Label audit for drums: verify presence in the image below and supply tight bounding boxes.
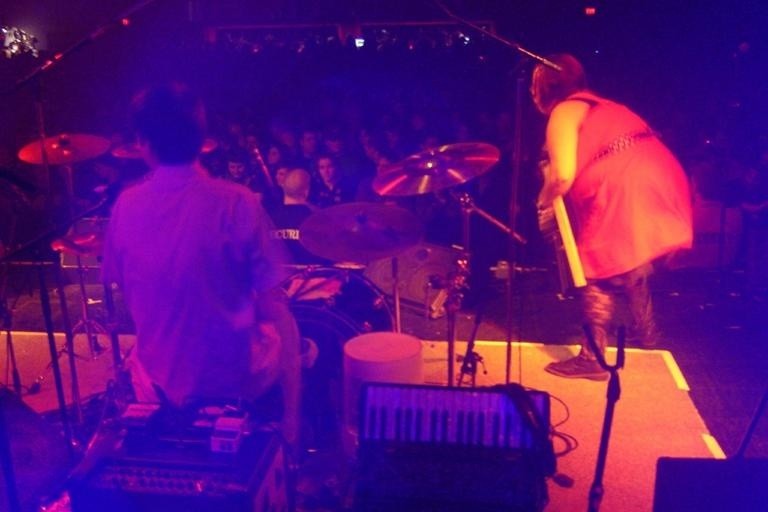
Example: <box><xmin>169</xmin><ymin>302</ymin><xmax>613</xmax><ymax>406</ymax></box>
<box><xmin>252</xmin><ymin>266</ymin><xmax>397</xmax><ymax>450</ymax></box>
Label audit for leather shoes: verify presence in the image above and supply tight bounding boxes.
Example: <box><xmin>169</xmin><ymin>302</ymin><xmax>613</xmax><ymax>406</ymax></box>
<box><xmin>545</xmin><ymin>356</ymin><xmax>609</xmax><ymax>381</ymax></box>
<box><xmin>613</xmin><ymin>324</ymin><xmax>659</xmax><ymax>347</ymax></box>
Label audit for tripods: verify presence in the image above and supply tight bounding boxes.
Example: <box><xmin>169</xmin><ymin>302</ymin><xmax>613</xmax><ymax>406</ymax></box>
<box><xmin>32</xmin><ymin>211</ymin><xmax>155</xmax><ymax>406</ymax></box>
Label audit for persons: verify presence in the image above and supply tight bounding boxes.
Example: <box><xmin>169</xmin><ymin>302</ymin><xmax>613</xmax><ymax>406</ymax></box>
<box><xmin>65</xmin><ymin>77</ymin><xmax>768</xmax><ymax>279</ymax></box>
<box><xmin>92</xmin><ymin>77</ymin><xmax>303</xmax><ymax>462</ymax></box>
<box><xmin>529</xmin><ymin>50</ymin><xmax>697</xmax><ymax>381</ymax></box>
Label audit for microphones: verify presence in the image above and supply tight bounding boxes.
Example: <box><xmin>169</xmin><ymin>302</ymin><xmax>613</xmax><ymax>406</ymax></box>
<box><xmin>90</xmin><ymin>1</ymin><xmax>141</xmax><ymax>46</ymax></box>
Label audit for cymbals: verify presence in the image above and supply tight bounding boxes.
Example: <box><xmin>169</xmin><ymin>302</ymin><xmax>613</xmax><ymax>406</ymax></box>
<box><xmin>298</xmin><ymin>201</ymin><xmax>425</xmax><ymax>263</ymax></box>
<box><xmin>17</xmin><ymin>133</ymin><xmax>111</xmax><ymax>165</ymax></box>
<box><xmin>371</xmin><ymin>141</ymin><xmax>503</xmax><ymax>197</ymax></box>
<box><xmin>112</xmin><ymin>137</ymin><xmax>218</xmax><ymax>159</ymax></box>
